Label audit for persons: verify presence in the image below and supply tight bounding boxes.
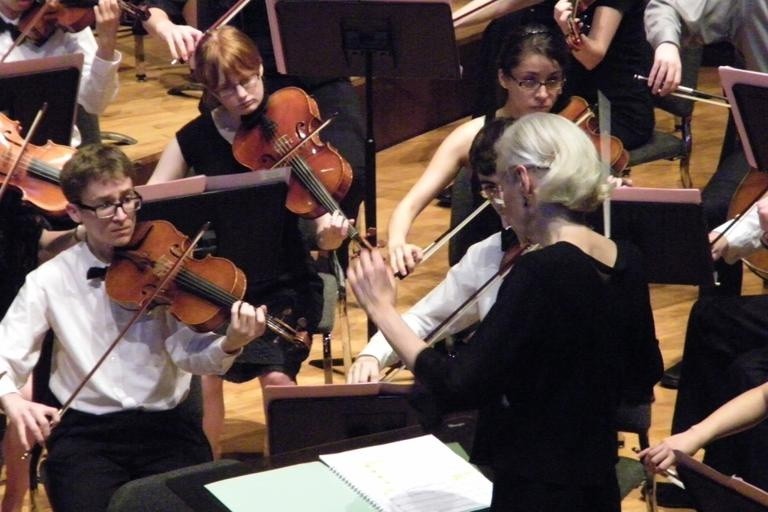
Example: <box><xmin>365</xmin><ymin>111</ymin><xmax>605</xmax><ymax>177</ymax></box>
<box><xmin>1</xmin><ymin>2</ymin><xmax>768</xmax><ymax>512</ymax></box>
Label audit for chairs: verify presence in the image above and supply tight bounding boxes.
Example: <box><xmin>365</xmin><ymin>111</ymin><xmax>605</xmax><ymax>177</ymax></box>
<box><xmin>621</xmin><ymin>45</ymin><xmax>700</xmax><ymax>189</ymax></box>
<box><xmin>308</xmin><ymin>249</ymin><xmax>341</xmax><ymax>387</ymax></box>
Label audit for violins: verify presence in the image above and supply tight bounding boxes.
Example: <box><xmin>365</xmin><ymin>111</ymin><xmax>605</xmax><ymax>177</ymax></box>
<box><xmin>551</xmin><ymin>95</ymin><xmax>630</xmax><ymax>175</ymax></box>
<box><xmin>19</xmin><ymin>1</ymin><xmax>150</xmax><ymax>42</ymax></box>
<box><xmin>105</xmin><ymin>219</ymin><xmax>312</xmax><ymax>350</ymax></box>
<box><xmin>0</xmin><ymin>111</ymin><xmax>78</xmax><ymax>217</ymax></box>
<box><xmin>231</xmin><ymin>86</ymin><xmax>373</xmax><ymax>249</ymax></box>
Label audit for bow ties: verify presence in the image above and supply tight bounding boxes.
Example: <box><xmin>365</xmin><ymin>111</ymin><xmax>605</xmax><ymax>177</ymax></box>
<box><xmin>0</xmin><ymin>18</ymin><xmax>24</xmax><ymax>43</ymax></box>
<box><xmin>87</xmin><ymin>267</ymin><xmax>109</xmax><ymax>279</ymax></box>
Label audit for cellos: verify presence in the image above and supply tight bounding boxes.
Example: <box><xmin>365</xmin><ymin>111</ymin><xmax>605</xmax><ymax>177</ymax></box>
<box><xmin>728</xmin><ymin>169</ymin><xmax>768</xmax><ymax>278</ymax></box>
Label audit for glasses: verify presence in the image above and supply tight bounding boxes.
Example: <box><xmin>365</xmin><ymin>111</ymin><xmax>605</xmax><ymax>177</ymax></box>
<box><xmin>510</xmin><ymin>76</ymin><xmax>566</xmax><ymax>88</ymax></box>
<box><xmin>78</xmin><ymin>192</ymin><xmax>142</xmax><ymax>218</ymax></box>
<box><xmin>212</xmin><ymin>73</ymin><xmax>259</xmax><ymax>97</ymax></box>
<box><xmin>479</xmin><ymin>184</ymin><xmax>502</xmax><ymax>199</ymax></box>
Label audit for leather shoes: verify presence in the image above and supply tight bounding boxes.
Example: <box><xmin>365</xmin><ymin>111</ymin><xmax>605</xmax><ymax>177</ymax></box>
<box><xmin>643</xmin><ymin>483</ymin><xmax>694</xmax><ymax>506</ymax></box>
<box><xmin>661</xmin><ymin>361</ymin><xmax>683</xmax><ymax>387</ymax></box>
